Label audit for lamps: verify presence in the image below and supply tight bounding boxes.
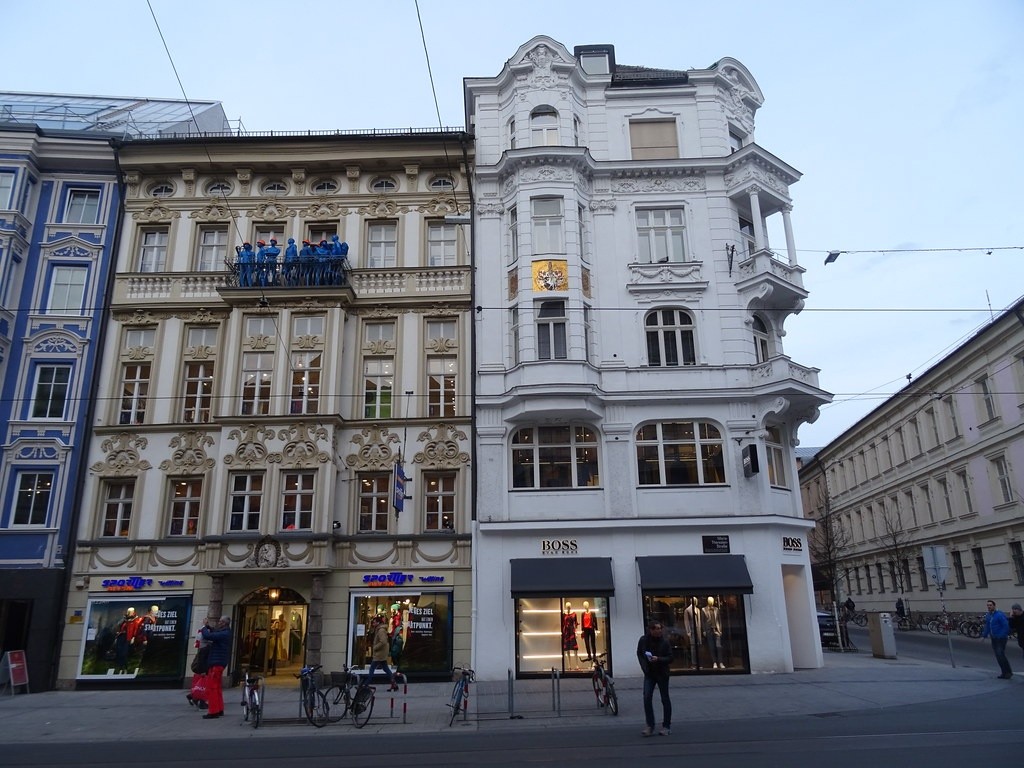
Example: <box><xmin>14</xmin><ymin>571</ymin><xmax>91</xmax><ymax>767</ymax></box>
<box><xmin>333</xmin><ymin>520</ymin><xmax>341</xmax><ymax>529</ymax></box>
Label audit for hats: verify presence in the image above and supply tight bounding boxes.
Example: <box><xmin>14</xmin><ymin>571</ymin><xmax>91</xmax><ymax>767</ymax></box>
<box><xmin>391</xmin><ymin>603</ymin><xmax>400</xmax><ymax>611</ymax></box>
<box><xmin>377</xmin><ymin>604</ymin><xmax>384</xmax><ymax>611</ymax></box>
<box><xmin>1012</xmin><ymin>603</ymin><xmax>1021</xmax><ymax>610</ymax></box>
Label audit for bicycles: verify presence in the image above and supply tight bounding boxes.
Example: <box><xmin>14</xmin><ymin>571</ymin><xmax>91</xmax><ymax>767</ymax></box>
<box><xmin>849</xmin><ymin>607</ymin><xmax>1018</xmax><ymax>640</ymax></box>
<box><xmin>446</xmin><ymin>662</ymin><xmax>477</xmax><ymax>728</ymax></box>
<box><xmin>323</xmin><ymin>663</ymin><xmax>376</xmax><ymax>729</ymax></box>
<box><xmin>292</xmin><ymin>662</ymin><xmax>330</xmax><ymax>728</ymax></box>
<box><xmin>232</xmin><ymin>665</ymin><xmax>261</xmax><ymax>729</ymax></box>
<box><xmin>580</xmin><ymin>648</ymin><xmax>619</xmax><ymax>717</ymax></box>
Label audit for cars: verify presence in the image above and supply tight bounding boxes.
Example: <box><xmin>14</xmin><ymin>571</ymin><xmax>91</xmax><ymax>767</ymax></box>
<box><xmin>815</xmin><ymin>609</ymin><xmax>849</xmax><ymax>647</ymax></box>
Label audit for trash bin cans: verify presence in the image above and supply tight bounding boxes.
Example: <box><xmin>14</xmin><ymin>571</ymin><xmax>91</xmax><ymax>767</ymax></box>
<box><xmin>867</xmin><ymin>611</ymin><xmax>897</xmax><ymax>659</ymax></box>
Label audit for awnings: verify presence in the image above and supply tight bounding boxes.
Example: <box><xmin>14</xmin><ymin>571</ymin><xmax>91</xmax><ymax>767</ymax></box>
<box><xmin>637</xmin><ymin>555</ymin><xmax>753</xmax><ymax>596</ymax></box>
<box><xmin>510</xmin><ymin>557</ymin><xmax>615</xmax><ymax>598</ymax></box>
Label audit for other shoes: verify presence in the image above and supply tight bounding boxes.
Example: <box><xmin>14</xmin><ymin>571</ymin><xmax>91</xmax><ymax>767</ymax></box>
<box><xmin>997</xmin><ymin>673</ymin><xmax>1012</xmax><ymax>679</ymax></box>
<box><xmin>187</xmin><ymin>694</ymin><xmax>193</xmax><ymax>705</ymax></box>
<box><xmin>387</xmin><ymin>686</ymin><xmax>399</xmax><ymax>692</ymax></box>
<box><xmin>203</xmin><ymin>713</ymin><xmax>219</xmax><ymax>718</ymax></box>
<box><xmin>200</xmin><ymin>704</ymin><xmax>208</xmax><ymax>709</ymax></box>
<box><xmin>219</xmin><ymin>711</ymin><xmax>224</xmax><ymax>716</ymax></box>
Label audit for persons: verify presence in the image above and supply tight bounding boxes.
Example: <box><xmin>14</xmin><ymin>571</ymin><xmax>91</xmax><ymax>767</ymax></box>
<box><xmin>367</xmin><ymin>617</ymin><xmax>399</xmax><ymax>692</ymax></box>
<box><xmin>701</xmin><ymin>597</ymin><xmax>725</xmax><ymax>669</ymax></box>
<box><xmin>185</xmin><ymin>616</ymin><xmax>232</xmax><ymax>719</ymax></box>
<box><xmin>1009</xmin><ymin>603</ymin><xmax>1024</xmax><ymax>649</ymax></box>
<box><xmin>896</xmin><ymin>598</ymin><xmax>906</xmax><ymax>626</ymax></box>
<box><xmin>637</xmin><ymin>620</ymin><xmax>673</xmax><ymax>735</ymax></box>
<box><xmin>239</xmin><ymin>235</ymin><xmax>348</xmax><ymax>287</ymax></box>
<box><xmin>115</xmin><ymin>605</ymin><xmax>159</xmax><ymax>674</ymax></box>
<box><xmin>563</xmin><ymin>602</ymin><xmax>580</xmax><ymax>670</ymax></box>
<box><xmin>581</xmin><ymin>601</ymin><xmax>598</xmax><ymax>658</ymax></box>
<box><xmin>684</xmin><ymin>597</ymin><xmax>703</xmax><ymax>669</ymax></box>
<box><xmin>845</xmin><ymin>597</ymin><xmax>855</xmax><ymax>621</ymax></box>
<box><xmin>979</xmin><ymin>600</ymin><xmax>1012</xmax><ymax>679</ymax></box>
<box><xmin>270</xmin><ymin>614</ymin><xmax>286</xmax><ymax>659</ymax></box>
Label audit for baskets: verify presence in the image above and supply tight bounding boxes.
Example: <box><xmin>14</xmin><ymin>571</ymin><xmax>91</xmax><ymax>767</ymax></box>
<box><xmin>331</xmin><ymin>671</ymin><xmax>346</xmax><ymax>686</ymax></box>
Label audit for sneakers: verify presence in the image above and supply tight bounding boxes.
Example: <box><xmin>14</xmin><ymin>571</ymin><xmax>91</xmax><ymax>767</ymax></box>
<box><xmin>659</xmin><ymin>728</ymin><xmax>672</xmax><ymax>736</ymax></box>
<box><xmin>642</xmin><ymin>726</ymin><xmax>654</xmax><ymax>736</ymax></box>
